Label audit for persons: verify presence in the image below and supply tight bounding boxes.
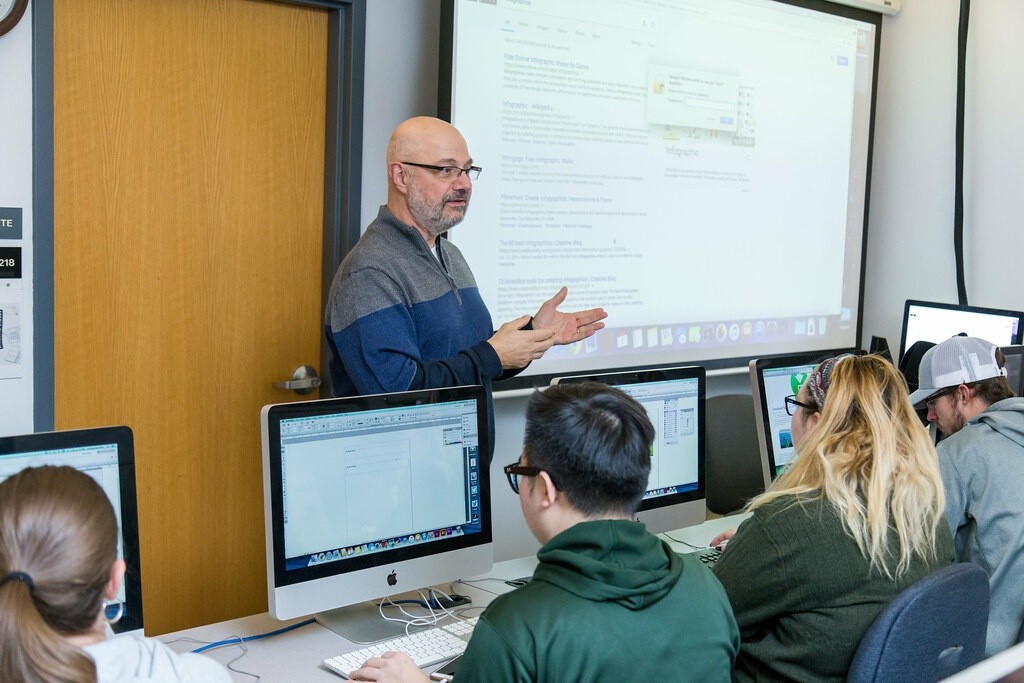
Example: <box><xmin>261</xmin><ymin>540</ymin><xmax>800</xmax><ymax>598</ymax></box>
<box><xmin>710</xmin><ymin>354</ymin><xmax>958</xmax><ymax>682</ymax></box>
<box><xmin>346</xmin><ymin>381</ymin><xmax>740</xmax><ymax>683</ymax></box>
<box><xmin>908</xmin><ymin>331</ymin><xmax>1024</xmax><ymax>656</ymax></box>
<box><xmin>323</xmin><ymin>115</ymin><xmax>608</xmax><ymax>397</ymax></box>
<box><xmin>0</xmin><ymin>464</ymin><xmax>235</xmax><ymax>682</ymax></box>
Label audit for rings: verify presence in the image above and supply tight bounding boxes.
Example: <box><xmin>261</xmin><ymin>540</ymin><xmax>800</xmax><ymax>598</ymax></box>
<box><xmin>577</xmin><ymin>329</ymin><xmax>579</xmax><ymax>333</ymax></box>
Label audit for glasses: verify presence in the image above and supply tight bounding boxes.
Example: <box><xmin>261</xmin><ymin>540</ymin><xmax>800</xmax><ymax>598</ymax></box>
<box><xmin>925</xmin><ymin>382</ymin><xmax>976</xmax><ymax>409</ymax></box>
<box><xmin>784</xmin><ymin>394</ymin><xmax>819</xmax><ymax>417</ymax></box>
<box><xmin>399</xmin><ymin>162</ymin><xmax>482</xmax><ymax>181</ymax></box>
<box><xmin>503</xmin><ymin>457</ymin><xmax>564</xmax><ymax>495</ymax></box>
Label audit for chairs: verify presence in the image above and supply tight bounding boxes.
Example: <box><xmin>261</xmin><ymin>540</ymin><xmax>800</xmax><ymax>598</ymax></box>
<box><xmin>845</xmin><ymin>562</ymin><xmax>990</xmax><ymax>683</ymax></box>
<box><xmin>703</xmin><ymin>394</ymin><xmax>765</xmax><ymax>514</ymax></box>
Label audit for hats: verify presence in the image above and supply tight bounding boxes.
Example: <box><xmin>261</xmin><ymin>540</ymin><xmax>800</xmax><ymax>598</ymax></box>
<box><xmin>905</xmin><ymin>334</ymin><xmax>1008</xmax><ymax>405</ymax></box>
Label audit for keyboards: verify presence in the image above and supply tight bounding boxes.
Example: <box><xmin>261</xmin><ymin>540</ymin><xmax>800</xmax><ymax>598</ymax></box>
<box><xmin>324</xmin><ymin>613</ymin><xmax>483</xmax><ymax>681</ymax></box>
<box><xmin>687</xmin><ymin>548</ymin><xmax>724</xmax><ymax>571</ymax></box>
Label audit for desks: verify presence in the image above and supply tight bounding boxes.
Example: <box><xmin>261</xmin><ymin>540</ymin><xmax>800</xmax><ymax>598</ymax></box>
<box><xmin>149</xmin><ymin>511</ymin><xmax>757</xmax><ymax>683</ymax></box>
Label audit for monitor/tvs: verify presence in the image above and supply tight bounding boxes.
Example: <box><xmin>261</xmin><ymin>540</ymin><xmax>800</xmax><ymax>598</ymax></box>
<box><xmin>550</xmin><ymin>365</ymin><xmax>707</xmax><ymax>536</ymax></box>
<box><xmin>260</xmin><ymin>384</ymin><xmax>494</xmax><ymax>645</ymax></box>
<box><xmin>749</xmin><ymin>350</ymin><xmax>869</xmax><ymax>491</ymax></box>
<box><xmin>898</xmin><ymin>300</ymin><xmax>1024</xmax><ymax>368</ymax></box>
<box><xmin>0</xmin><ymin>425</ymin><xmax>145</xmax><ymax>639</ymax></box>
